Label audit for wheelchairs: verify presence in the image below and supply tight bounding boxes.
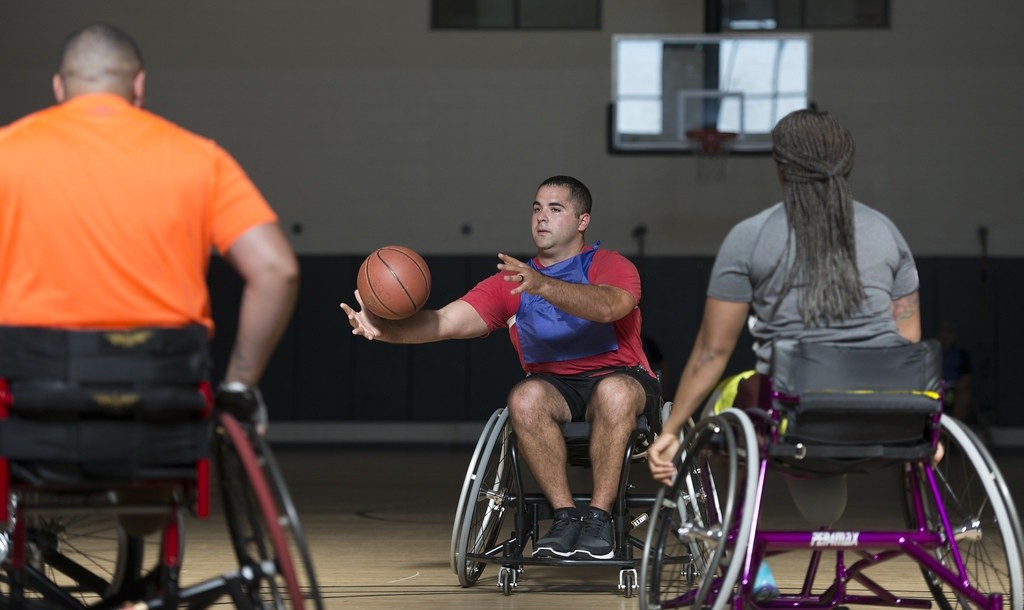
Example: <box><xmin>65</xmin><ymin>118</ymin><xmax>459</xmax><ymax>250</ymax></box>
<box><xmin>451</xmin><ymin>368</ymin><xmax>729</xmax><ymax>586</ymax></box>
<box><xmin>640</xmin><ymin>338</ymin><xmax>1024</xmax><ymax>610</ymax></box>
<box><xmin>0</xmin><ymin>321</ymin><xmax>326</xmax><ymax>610</ymax></box>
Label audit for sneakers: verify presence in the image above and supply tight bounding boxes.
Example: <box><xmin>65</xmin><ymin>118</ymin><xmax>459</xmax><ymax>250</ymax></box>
<box><xmin>744</xmin><ymin>557</ymin><xmax>781</xmax><ymax>600</ymax></box>
<box><xmin>568</xmin><ymin>512</ymin><xmax>618</xmax><ymax>560</ymax></box>
<box><xmin>531</xmin><ymin>508</ymin><xmax>582</xmax><ymax>559</ymax></box>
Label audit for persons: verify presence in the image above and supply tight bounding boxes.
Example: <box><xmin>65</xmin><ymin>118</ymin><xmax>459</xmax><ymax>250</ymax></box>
<box><xmin>642</xmin><ymin>106</ymin><xmax>924</xmax><ymax>596</ymax></box>
<box><xmin>0</xmin><ymin>18</ymin><xmax>298</xmax><ymax>432</ymax></box>
<box><xmin>338</xmin><ymin>174</ymin><xmax>665</xmax><ymax>567</ymax></box>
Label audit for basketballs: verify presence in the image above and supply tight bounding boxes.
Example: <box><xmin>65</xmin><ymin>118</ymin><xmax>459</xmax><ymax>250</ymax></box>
<box><xmin>357</xmin><ymin>245</ymin><xmax>432</xmax><ymax>321</ymax></box>
